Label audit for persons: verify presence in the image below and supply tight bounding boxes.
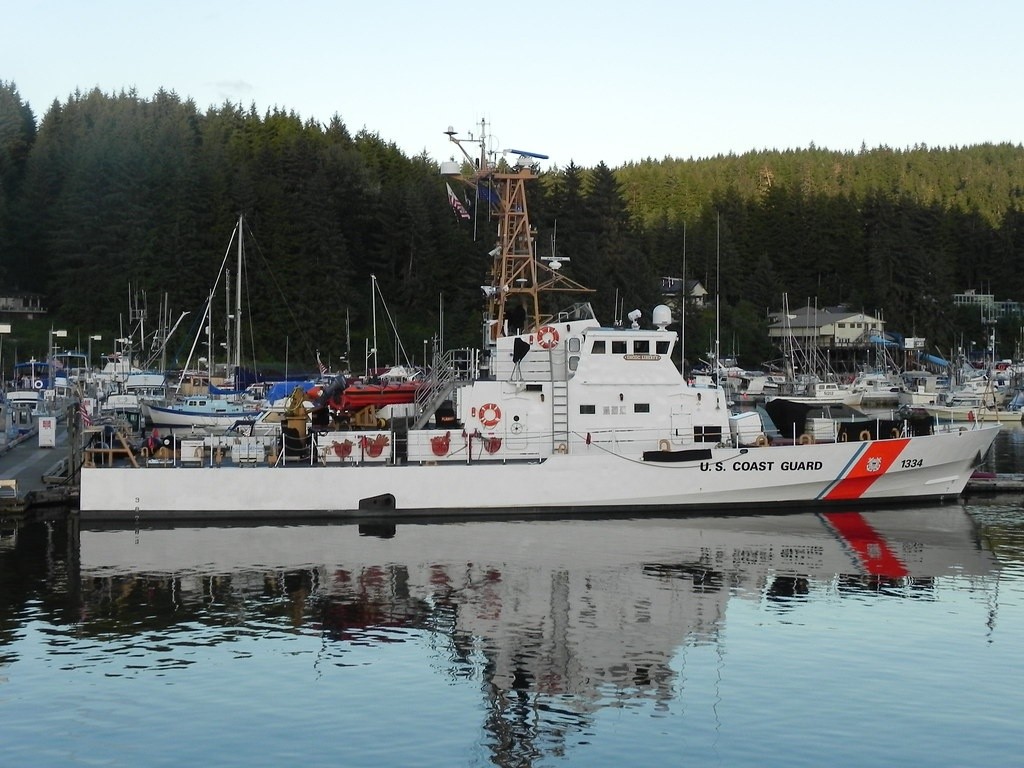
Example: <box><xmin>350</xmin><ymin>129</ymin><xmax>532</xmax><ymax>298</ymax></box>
<box><xmin>509</xmin><ymin>303</ymin><xmax>527</xmax><ymax>335</ymax></box>
<box><xmin>140</xmin><ymin>414</ymin><xmax>146</xmax><ymax>439</ymax></box>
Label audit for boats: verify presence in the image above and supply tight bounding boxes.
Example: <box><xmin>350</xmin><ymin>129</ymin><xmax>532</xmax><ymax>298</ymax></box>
<box><xmin>77</xmin><ymin>289</ymin><xmax>1005</xmax><ymax>523</ymax></box>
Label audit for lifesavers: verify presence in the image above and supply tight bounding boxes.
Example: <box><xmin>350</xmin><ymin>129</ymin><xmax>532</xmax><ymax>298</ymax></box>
<box><xmin>890</xmin><ymin>427</ymin><xmax>899</xmax><ymax>439</ymax></box>
<box><xmin>479</xmin><ymin>403</ymin><xmax>501</xmax><ymax>426</ymax></box>
<box><xmin>859</xmin><ymin>430</ymin><xmax>871</xmax><ymax>441</ymax></box>
<box><xmin>756</xmin><ymin>435</ymin><xmax>768</xmax><ymax>448</ymax></box>
<box><xmin>799</xmin><ymin>433</ymin><xmax>811</xmax><ymax>445</ymax></box>
<box><xmin>658</xmin><ymin>439</ymin><xmax>670</xmax><ymax>451</ymax></box>
<box><xmin>537</xmin><ymin>326</ymin><xmax>560</xmax><ymax>349</ymax></box>
<box><xmin>34</xmin><ymin>380</ymin><xmax>43</xmax><ymax>389</ymax></box>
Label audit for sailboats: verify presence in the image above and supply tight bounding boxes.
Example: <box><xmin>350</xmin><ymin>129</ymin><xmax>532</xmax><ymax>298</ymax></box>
<box><xmin>0</xmin><ymin>116</ymin><xmax>1023</xmax><ymax>488</ymax></box>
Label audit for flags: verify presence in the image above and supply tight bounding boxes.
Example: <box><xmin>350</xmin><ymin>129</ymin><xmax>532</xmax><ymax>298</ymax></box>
<box><xmin>78</xmin><ymin>394</ymin><xmax>93</xmax><ymax>426</ymax></box>
